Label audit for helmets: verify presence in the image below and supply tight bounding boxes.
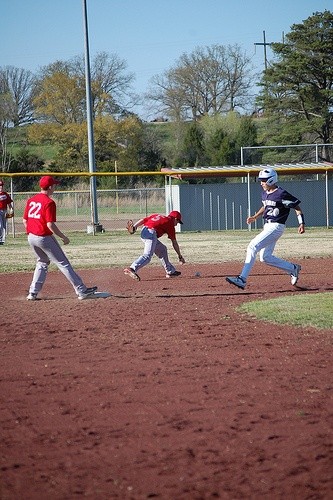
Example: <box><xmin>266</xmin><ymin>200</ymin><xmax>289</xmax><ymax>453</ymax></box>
<box><xmin>258</xmin><ymin>169</ymin><xmax>279</xmax><ymax>186</ymax></box>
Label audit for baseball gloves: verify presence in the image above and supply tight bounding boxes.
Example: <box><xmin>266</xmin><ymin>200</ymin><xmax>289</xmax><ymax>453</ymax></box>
<box><xmin>125</xmin><ymin>220</ymin><xmax>137</xmax><ymax>234</ymax></box>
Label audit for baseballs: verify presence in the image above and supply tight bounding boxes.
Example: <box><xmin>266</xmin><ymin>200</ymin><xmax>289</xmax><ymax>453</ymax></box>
<box><xmin>195</xmin><ymin>272</ymin><xmax>201</xmax><ymax>277</ymax></box>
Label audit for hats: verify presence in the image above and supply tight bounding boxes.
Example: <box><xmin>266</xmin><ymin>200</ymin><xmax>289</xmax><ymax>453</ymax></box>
<box><xmin>39</xmin><ymin>176</ymin><xmax>62</xmax><ymax>190</ymax></box>
<box><xmin>170</xmin><ymin>211</ymin><xmax>183</xmax><ymax>225</ymax></box>
<box><xmin>0</xmin><ymin>181</ymin><xmax>4</xmax><ymax>186</ymax></box>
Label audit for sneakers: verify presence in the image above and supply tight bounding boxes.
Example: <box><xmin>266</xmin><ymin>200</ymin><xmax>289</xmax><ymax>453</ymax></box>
<box><xmin>166</xmin><ymin>271</ymin><xmax>181</xmax><ymax>278</ymax></box>
<box><xmin>78</xmin><ymin>286</ymin><xmax>98</xmax><ymax>299</ymax></box>
<box><xmin>291</xmin><ymin>265</ymin><xmax>302</xmax><ymax>286</ymax></box>
<box><xmin>225</xmin><ymin>277</ymin><xmax>247</xmax><ymax>290</ymax></box>
<box><xmin>123</xmin><ymin>267</ymin><xmax>140</xmax><ymax>281</ymax></box>
<box><xmin>25</xmin><ymin>293</ymin><xmax>37</xmax><ymax>300</ymax></box>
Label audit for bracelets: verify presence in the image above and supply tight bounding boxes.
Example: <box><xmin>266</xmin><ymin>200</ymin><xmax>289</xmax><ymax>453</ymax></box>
<box><xmin>179</xmin><ymin>255</ymin><xmax>183</xmax><ymax>259</ymax></box>
<box><xmin>253</xmin><ymin>215</ymin><xmax>257</xmax><ymax>219</ymax></box>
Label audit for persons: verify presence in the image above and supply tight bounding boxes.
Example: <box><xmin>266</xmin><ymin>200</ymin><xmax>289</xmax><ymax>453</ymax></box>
<box><xmin>23</xmin><ymin>176</ymin><xmax>98</xmax><ymax>300</ymax></box>
<box><xmin>123</xmin><ymin>210</ymin><xmax>186</xmax><ymax>281</ymax></box>
<box><xmin>0</xmin><ymin>179</ymin><xmax>14</xmax><ymax>245</ymax></box>
<box><xmin>226</xmin><ymin>168</ymin><xmax>306</xmax><ymax>290</ymax></box>
